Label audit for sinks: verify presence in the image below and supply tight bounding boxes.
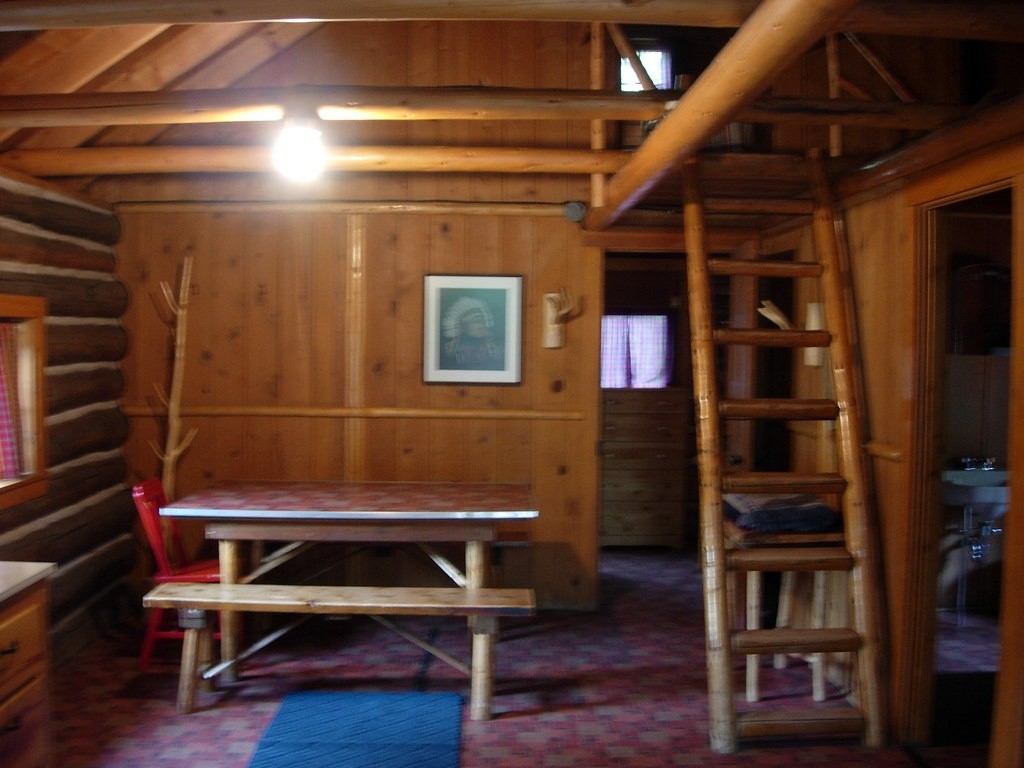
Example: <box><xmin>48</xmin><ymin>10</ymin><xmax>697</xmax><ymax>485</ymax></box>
<box><xmin>929</xmin><ymin>470</ymin><xmax>1011</xmax><ymax>522</ymax></box>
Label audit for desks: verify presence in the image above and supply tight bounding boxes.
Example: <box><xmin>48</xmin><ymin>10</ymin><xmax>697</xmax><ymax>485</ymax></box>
<box><xmin>158</xmin><ymin>481</ymin><xmax>542</xmax><ymax>683</ymax></box>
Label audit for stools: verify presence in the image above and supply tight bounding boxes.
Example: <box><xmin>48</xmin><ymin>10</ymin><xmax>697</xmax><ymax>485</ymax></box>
<box><xmin>720</xmin><ymin>494</ymin><xmax>847</xmax><ymax>704</ymax></box>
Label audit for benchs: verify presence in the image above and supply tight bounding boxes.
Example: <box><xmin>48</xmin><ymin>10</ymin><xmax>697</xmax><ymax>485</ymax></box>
<box><xmin>305</xmin><ymin>531</ymin><xmax>534</xmax><ymax>582</ymax></box>
<box><xmin>144</xmin><ymin>581</ymin><xmax>536</xmax><ymax>721</ymax></box>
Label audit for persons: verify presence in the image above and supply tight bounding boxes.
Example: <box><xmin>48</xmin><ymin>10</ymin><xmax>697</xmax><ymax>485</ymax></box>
<box><xmin>936</xmin><ymin>458</ymin><xmax>1005</xmax><ymax>601</ymax></box>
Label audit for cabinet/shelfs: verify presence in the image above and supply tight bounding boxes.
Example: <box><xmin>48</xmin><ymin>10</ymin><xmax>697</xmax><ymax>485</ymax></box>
<box><xmin>0</xmin><ymin>577</ymin><xmax>55</xmax><ymax>768</ymax></box>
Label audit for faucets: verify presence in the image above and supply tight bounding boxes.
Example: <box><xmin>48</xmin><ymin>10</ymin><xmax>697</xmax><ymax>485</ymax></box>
<box><xmin>959</xmin><ymin>454</ymin><xmax>998</xmax><ymax>472</ymax></box>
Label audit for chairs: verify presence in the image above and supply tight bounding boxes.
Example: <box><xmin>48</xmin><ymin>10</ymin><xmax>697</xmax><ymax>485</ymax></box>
<box><xmin>133</xmin><ymin>478</ymin><xmax>245</xmax><ymax>673</ymax></box>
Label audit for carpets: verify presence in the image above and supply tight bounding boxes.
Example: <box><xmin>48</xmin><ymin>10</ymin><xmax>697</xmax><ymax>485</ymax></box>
<box><xmin>247</xmin><ymin>689</ymin><xmax>463</xmax><ymax>768</ymax></box>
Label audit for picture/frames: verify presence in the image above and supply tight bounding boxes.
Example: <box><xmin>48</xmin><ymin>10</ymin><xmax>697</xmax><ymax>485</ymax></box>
<box><xmin>422</xmin><ymin>274</ymin><xmax>523</xmax><ymax>385</ymax></box>
